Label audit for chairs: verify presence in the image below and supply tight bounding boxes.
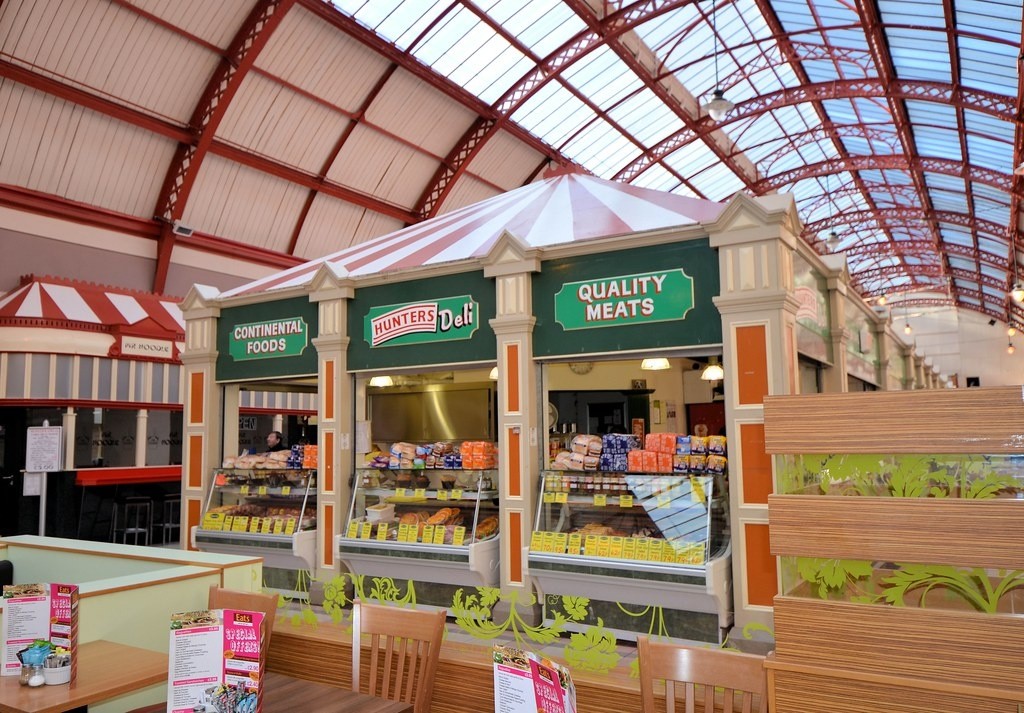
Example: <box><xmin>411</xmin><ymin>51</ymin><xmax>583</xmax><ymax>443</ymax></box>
<box><xmin>350</xmin><ymin>597</ymin><xmax>447</xmax><ymax>713</ymax></box>
<box><xmin>637</xmin><ymin>636</ymin><xmax>768</xmax><ymax>713</ymax></box>
<box><xmin>207</xmin><ymin>584</ymin><xmax>281</xmax><ymax>655</ymax></box>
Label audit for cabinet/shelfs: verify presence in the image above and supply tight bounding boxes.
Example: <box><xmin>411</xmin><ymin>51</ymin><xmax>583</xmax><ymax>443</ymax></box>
<box><xmin>194</xmin><ymin>466</ymin><xmax>316</xmax><ymax>600</ymax></box>
<box><xmin>334</xmin><ymin>466</ymin><xmax>500</xmax><ymax>622</ymax></box>
<box><xmin>527</xmin><ymin>469</ymin><xmax>733</xmax><ymax>650</ymax></box>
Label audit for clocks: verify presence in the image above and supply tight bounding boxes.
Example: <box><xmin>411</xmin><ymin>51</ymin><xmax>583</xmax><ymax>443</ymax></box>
<box><xmin>569</xmin><ymin>362</ymin><xmax>593</xmax><ymax>375</ymax></box>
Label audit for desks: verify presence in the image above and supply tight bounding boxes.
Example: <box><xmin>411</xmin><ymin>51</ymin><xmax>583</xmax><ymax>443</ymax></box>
<box><xmin>0</xmin><ymin>638</ymin><xmax>414</xmax><ymax>713</ymax></box>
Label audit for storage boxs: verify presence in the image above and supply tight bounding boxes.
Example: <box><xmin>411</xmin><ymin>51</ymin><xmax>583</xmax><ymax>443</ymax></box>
<box><xmin>598</xmin><ymin>433</ymin><xmax>691</xmax><ymax>473</ymax></box>
<box><xmin>152</xmin><ymin>523</ymin><xmax>180</xmax><ymax>544</ymax></box>
<box><xmin>115</xmin><ymin>529</ymin><xmax>150</xmax><ymax>547</ymax></box>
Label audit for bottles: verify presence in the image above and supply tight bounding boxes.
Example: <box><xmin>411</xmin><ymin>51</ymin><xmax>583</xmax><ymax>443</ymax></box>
<box><xmin>28</xmin><ymin>663</ymin><xmax>45</xmax><ymax>687</ymax></box>
<box><xmin>19</xmin><ymin>662</ymin><xmax>33</xmax><ymax>686</ymax></box>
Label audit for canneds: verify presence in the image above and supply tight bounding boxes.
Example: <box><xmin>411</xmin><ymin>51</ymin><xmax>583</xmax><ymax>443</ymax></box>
<box><xmin>544</xmin><ymin>475</ymin><xmax>693</xmax><ymax>499</ymax></box>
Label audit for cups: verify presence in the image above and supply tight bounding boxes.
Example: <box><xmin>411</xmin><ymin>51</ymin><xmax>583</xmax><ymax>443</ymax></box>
<box><xmin>43</xmin><ymin>665</ymin><xmax>71</xmax><ymax>685</ymax></box>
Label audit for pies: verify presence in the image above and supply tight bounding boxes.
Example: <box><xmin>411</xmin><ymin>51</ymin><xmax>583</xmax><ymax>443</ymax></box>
<box><xmin>399</xmin><ymin>507</ymin><xmax>498</xmax><ymax>538</ymax></box>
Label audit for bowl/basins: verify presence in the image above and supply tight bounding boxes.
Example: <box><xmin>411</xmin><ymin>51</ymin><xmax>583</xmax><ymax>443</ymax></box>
<box><xmin>365</xmin><ymin>503</ymin><xmax>395</xmax><ymax>525</ymax></box>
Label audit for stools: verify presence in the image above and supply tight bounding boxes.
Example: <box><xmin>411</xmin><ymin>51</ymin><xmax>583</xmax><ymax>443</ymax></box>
<box><xmin>162</xmin><ymin>494</ymin><xmax>181</xmax><ymax>545</ymax></box>
<box><xmin>108</xmin><ymin>496</ymin><xmax>153</xmax><ymax>546</ymax></box>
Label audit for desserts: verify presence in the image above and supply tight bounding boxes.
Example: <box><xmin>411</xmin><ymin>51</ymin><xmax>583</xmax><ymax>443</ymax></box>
<box><xmin>393</xmin><ymin>473</ymin><xmax>456</xmax><ymax>488</ymax></box>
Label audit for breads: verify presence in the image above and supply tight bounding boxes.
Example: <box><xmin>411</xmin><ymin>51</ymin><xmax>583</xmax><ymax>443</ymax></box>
<box><xmin>222</xmin><ymin>450</ymin><xmax>291</xmax><ymax>469</ymax></box>
<box><xmin>206</xmin><ymin>503</ymin><xmax>317</xmax><ymax>532</ymax></box>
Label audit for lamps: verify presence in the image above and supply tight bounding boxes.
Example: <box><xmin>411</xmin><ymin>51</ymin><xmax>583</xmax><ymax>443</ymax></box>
<box><xmin>640</xmin><ymin>358</ymin><xmax>671</xmax><ymax>371</ymax></box>
<box><xmin>824</xmin><ymin>175</ymin><xmax>844</xmax><ymax>249</ymax></box>
<box><xmin>488</xmin><ymin>367</ymin><xmax>499</xmax><ymax>380</ymax></box>
<box><xmin>1007</xmin><ymin>231</ymin><xmax>1024</xmax><ymax>304</ymax></box>
<box><xmin>369</xmin><ymin>376</ymin><xmax>394</xmax><ymax>389</ymax></box>
<box><xmin>153</xmin><ymin>216</ymin><xmax>195</xmax><ymax>238</ymax></box>
<box><xmin>1006</xmin><ymin>311</ymin><xmax>1016</xmax><ymax>337</ymax></box>
<box><xmin>1006</xmin><ymin>337</ymin><xmax>1016</xmax><ymax>355</ymax></box>
<box><xmin>699</xmin><ymin>357</ymin><xmax>724</xmax><ymax>381</ymax></box>
<box><xmin>904</xmin><ymin>292</ymin><xmax>912</xmax><ymax>334</ymax></box>
<box><xmin>702</xmin><ymin>0</ymin><xmax>736</xmax><ymax>121</ymax></box>
<box><xmin>878</xmin><ymin>253</ymin><xmax>888</xmax><ymax>305</ymax></box>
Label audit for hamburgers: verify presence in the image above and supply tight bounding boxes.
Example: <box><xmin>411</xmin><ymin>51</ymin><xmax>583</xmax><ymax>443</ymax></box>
<box><xmin>182</xmin><ymin>610</ymin><xmax>220</xmax><ymax>628</ymax></box>
<box><xmin>13</xmin><ymin>584</ymin><xmax>46</xmax><ymax>597</ymax></box>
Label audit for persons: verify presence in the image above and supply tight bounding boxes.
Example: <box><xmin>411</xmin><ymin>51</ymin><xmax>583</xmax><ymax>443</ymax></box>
<box><xmin>266</xmin><ymin>431</ymin><xmax>288</xmax><ymax>452</ymax></box>
<box><xmin>299</xmin><ymin>437</ymin><xmax>310</xmax><ymax>445</ymax></box>
